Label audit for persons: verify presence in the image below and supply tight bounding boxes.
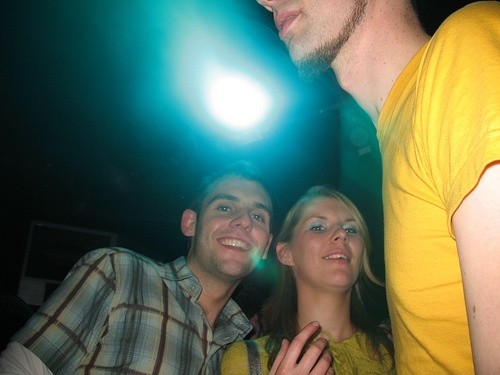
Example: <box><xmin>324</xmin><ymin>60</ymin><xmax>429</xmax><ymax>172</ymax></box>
<box><xmin>256</xmin><ymin>0</ymin><xmax>500</xmax><ymax>375</ymax></box>
<box><xmin>221</xmin><ymin>184</ymin><xmax>396</xmax><ymax>374</ymax></box>
<box><xmin>0</xmin><ymin>162</ymin><xmax>272</xmax><ymax>375</ymax></box>
